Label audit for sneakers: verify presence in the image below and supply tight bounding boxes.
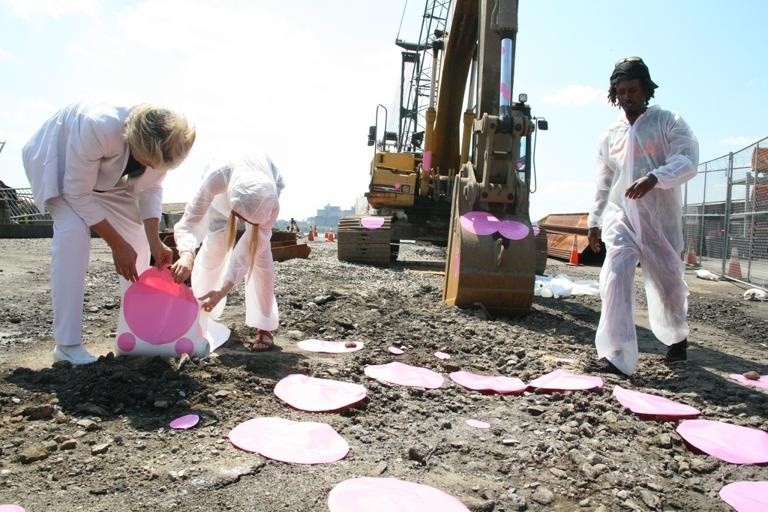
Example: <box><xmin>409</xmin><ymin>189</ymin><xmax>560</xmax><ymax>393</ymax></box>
<box><xmin>583</xmin><ymin>357</ymin><xmax>620</xmax><ymax>373</ymax></box>
<box><xmin>665</xmin><ymin>340</ymin><xmax>688</xmax><ymax>364</ymax></box>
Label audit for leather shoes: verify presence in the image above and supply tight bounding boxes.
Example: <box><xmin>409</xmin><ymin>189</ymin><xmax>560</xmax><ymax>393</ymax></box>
<box><xmin>53</xmin><ymin>343</ymin><xmax>97</xmax><ymax>366</ymax></box>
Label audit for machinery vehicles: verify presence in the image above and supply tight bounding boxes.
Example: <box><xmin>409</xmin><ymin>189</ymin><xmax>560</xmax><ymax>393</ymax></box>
<box><xmin>337</xmin><ymin>0</ymin><xmax>547</xmax><ymax>316</ymax></box>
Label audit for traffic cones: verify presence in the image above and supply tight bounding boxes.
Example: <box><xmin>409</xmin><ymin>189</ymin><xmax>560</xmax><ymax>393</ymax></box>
<box><xmin>566</xmin><ymin>235</ymin><xmax>582</xmax><ymax>265</ymax></box>
<box><xmin>309</xmin><ymin>224</ymin><xmax>317</xmax><ymax>241</ymax></box>
<box><xmin>324</xmin><ymin>225</ymin><xmax>333</xmax><ymax>241</ymax></box>
<box><xmin>728</xmin><ymin>246</ymin><xmax>744</xmax><ymax>280</ymax></box>
<box><xmin>683</xmin><ymin>235</ymin><xmax>700</xmax><ymax>267</ymax></box>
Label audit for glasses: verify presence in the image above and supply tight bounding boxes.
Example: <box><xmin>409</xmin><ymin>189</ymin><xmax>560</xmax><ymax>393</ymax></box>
<box><xmin>615</xmin><ymin>55</ymin><xmax>641</xmax><ymax>66</ymax></box>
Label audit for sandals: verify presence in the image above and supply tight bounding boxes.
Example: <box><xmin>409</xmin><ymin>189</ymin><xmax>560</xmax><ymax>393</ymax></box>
<box><xmin>253</xmin><ymin>330</ymin><xmax>274</xmax><ymax>353</ymax></box>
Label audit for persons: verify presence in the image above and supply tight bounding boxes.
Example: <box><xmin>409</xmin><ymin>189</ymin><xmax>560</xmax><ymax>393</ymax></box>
<box><xmin>581</xmin><ymin>57</ymin><xmax>698</xmax><ymax>377</ymax></box>
<box><xmin>22</xmin><ymin>99</ymin><xmax>197</xmax><ymax>366</ymax></box>
<box><xmin>171</xmin><ymin>154</ymin><xmax>287</xmax><ymax>351</ymax></box>
